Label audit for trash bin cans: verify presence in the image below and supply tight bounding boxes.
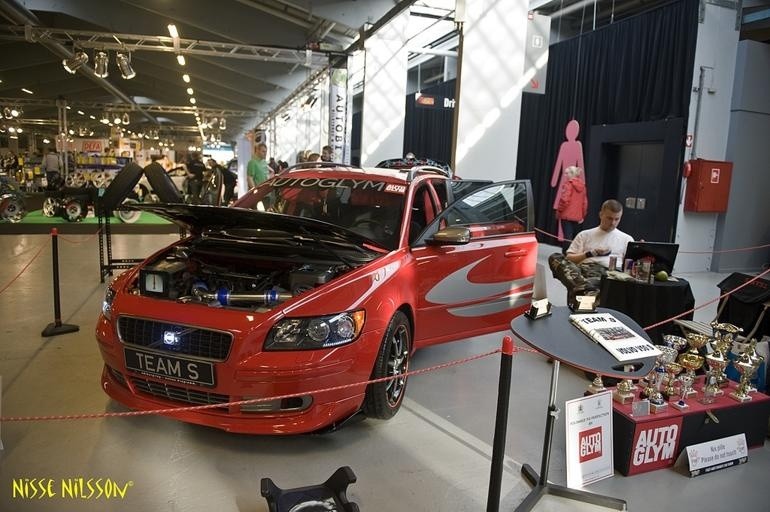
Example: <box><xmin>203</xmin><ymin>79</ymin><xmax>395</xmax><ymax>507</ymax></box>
<box><xmin>716</xmin><ymin>271</ymin><xmax>770</xmax><ymax>342</ymax></box>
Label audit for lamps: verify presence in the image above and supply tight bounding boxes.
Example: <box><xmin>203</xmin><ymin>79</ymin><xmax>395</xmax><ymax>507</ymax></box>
<box><xmin>61</xmin><ymin>39</ymin><xmax>137</xmax><ymax>80</ymax></box>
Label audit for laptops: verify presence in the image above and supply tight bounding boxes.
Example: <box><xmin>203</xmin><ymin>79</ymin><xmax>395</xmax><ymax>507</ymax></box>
<box><xmin>605</xmin><ymin>241</ymin><xmax>679</xmax><ymax>277</ymax></box>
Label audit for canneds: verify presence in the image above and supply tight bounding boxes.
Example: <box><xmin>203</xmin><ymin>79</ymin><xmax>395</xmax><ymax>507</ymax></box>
<box><xmin>624</xmin><ymin>258</ymin><xmax>633</xmax><ymax>274</ymax></box>
<box><xmin>609</xmin><ymin>255</ymin><xmax>616</xmax><ymax>270</ymax></box>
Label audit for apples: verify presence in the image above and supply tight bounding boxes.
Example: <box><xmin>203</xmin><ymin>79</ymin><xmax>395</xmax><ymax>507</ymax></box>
<box><xmin>656</xmin><ymin>271</ymin><xmax>668</xmax><ymax>281</ymax></box>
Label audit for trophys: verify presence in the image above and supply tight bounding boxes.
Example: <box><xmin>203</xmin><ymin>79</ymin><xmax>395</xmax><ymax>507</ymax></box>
<box><xmin>588</xmin><ymin>321</ymin><xmax>763</xmax><ymax>414</ymax></box>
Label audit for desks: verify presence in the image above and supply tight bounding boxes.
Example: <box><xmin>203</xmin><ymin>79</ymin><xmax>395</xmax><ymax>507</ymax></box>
<box><xmin>509</xmin><ymin>269</ymin><xmax>693</xmax><ymax>512</ymax></box>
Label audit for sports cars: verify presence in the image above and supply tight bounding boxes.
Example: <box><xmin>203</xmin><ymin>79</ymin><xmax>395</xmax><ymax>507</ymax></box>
<box><xmin>95</xmin><ymin>159</ymin><xmax>537</xmax><ymax>439</ymax></box>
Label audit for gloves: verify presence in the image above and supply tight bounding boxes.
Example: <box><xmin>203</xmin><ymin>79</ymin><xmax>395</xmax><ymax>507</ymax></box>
<box><xmin>586</xmin><ymin>247</ymin><xmax>611</xmax><ymax>258</ymax></box>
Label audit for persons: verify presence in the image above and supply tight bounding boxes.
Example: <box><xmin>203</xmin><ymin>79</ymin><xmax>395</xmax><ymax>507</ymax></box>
<box><xmin>42</xmin><ymin>148</ymin><xmax>63</xmax><ymax>186</ymax></box>
<box><xmin>548</xmin><ymin>200</ymin><xmax>636</xmax><ymax>305</ymax></box>
<box><xmin>190</xmin><ymin>152</ymin><xmax>237</xmax><ymax>206</ymax></box>
<box><xmin>558</xmin><ymin>166</ymin><xmax>588</xmax><ymax>255</ymax></box>
<box><xmin>5</xmin><ymin>151</ymin><xmax>18</xmax><ymax>174</ymax></box>
<box><xmin>247</xmin><ymin>144</ymin><xmax>332</xmax><ymax>210</ymax></box>
